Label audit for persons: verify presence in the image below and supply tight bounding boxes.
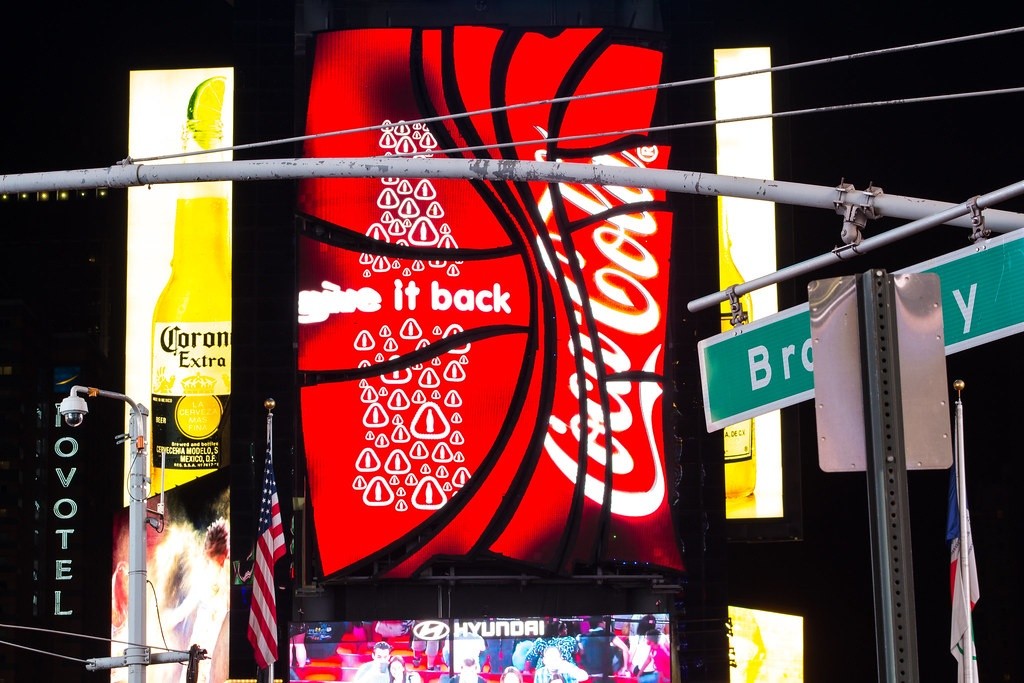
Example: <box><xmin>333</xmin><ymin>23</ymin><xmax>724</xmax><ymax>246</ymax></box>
<box><xmin>290</xmin><ymin>623</ymin><xmax>308</xmax><ymax>667</ymax></box>
<box><xmin>166</xmin><ymin>518</ymin><xmax>230</xmax><ymax>683</ymax></box>
<box><xmin>524</xmin><ymin>614</ymin><xmax>670</xmax><ymax>683</ymax></box>
<box><xmin>353</xmin><ymin>642</ymin><xmax>420</xmax><ymax>683</ymax></box>
<box><xmin>443</xmin><ymin>620</ymin><xmax>486</xmax><ymax>683</ymax></box>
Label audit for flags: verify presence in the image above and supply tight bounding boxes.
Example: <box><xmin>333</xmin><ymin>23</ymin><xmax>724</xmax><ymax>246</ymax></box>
<box><xmin>248</xmin><ymin>415</ymin><xmax>287</xmax><ymax>669</ymax></box>
<box><xmin>947</xmin><ymin>411</ymin><xmax>979</xmax><ymax>683</ymax></box>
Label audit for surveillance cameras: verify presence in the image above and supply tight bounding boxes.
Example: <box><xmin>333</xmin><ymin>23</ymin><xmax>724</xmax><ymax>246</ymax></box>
<box><xmin>61</xmin><ymin>397</ymin><xmax>89</xmax><ymax>427</ymax></box>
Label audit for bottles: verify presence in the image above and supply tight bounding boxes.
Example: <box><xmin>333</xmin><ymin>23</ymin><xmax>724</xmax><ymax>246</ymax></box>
<box><xmin>149</xmin><ymin>78</ymin><xmax>232</xmax><ymax>495</ymax></box>
<box><xmin>716</xmin><ymin>141</ymin><xmax>757</xmax><ymax>498</ymax></box>
